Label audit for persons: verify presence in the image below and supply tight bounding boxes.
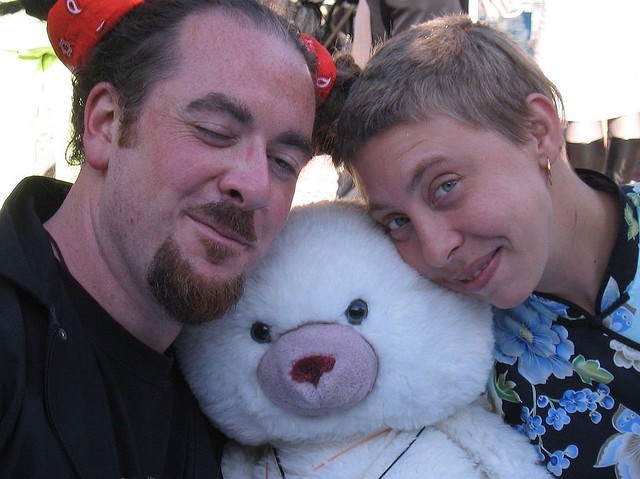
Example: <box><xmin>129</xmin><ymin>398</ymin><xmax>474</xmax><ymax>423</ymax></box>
<box><xmin>334</xmin><ymin>14</ymin><xmax>638</xmax><ymax>478</ymax></box>
<box><xmin>0</xmin><ymin>1</ymin><xmax>360</xmax><ymax>479</ymax></box>
<box><xmin>338</xmin><ymin>1</ymin><xmax>468</xmax><ymax>198</ymax></box>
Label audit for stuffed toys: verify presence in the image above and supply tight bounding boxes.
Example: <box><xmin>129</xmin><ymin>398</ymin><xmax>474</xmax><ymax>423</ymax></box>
<box><xmin>173</xmin><ymin>198</ymin><xmax>553</xmax><ymax>478</ymax></box>
